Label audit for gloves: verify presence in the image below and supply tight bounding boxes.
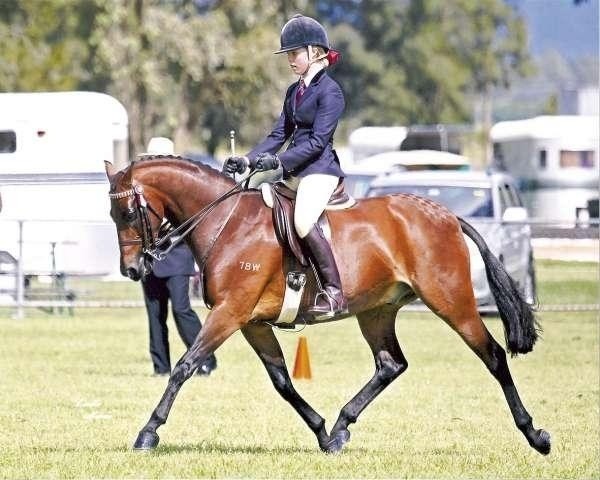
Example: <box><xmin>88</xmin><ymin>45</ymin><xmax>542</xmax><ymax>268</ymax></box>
<box><xmin>256</xmin><ymin>152</ymin><xmax>279</xmax><ymax>172</ymax></box>
<box><xmin>223</xmin><ymin>157</ymin><xmax>247</xmax><ymax>176</ymax></box>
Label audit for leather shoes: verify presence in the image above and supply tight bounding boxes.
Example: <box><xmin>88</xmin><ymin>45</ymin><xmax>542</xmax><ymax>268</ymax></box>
<box><xmin>197</xmin><ymin>355</ymin><xmax>216</xmax><ymax>375</ymax></box>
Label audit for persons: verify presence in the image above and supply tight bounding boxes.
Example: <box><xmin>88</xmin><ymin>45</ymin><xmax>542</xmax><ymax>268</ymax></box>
<box><xmin>141</xmin><ymin>137</ymin><xmax>216</xmax><ymax>378</ymax></box>
<box><xmin>222</xmin><ymin>14</ymin><xmax>349</xmax><ymax>315</ymax></box>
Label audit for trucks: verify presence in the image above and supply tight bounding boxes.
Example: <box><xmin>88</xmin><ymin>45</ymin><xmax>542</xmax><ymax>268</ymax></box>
<box><xmin>0</xmin><ymin>91</ymin><xmax>131</xmax><ymax>294</ymax></box>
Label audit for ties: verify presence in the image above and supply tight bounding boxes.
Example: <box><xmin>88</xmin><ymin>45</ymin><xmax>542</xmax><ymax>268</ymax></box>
<box><xmin>296</xmin><ymin>80</ymin><xmax>305</xmax><ymax>102</ymax></box>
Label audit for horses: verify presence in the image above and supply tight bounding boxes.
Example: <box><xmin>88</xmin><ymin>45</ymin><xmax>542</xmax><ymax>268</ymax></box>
<box><xmin>103</xmin><ymin>155</ymin><xmax>552</xmax><ymax>457</ymax></box>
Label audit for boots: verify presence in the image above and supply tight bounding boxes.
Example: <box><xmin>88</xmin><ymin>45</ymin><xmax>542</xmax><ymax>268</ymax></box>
<box><xmin>298</xmin><ymin>223</ymin><xmax>347</xmax><ymax>315</ymax></box>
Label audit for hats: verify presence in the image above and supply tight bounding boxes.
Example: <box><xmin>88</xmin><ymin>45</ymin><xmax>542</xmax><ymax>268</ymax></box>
<box><xmin>137</xmin><ymin>137</ymin><xmax>181</xmax><ymax>157</ymax></box>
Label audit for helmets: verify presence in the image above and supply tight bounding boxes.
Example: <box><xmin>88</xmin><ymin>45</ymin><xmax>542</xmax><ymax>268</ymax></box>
<box><xmin>274</xmin><ymin>14</ymin><xmax>330</xmax><ymax>54</ymax></box>
